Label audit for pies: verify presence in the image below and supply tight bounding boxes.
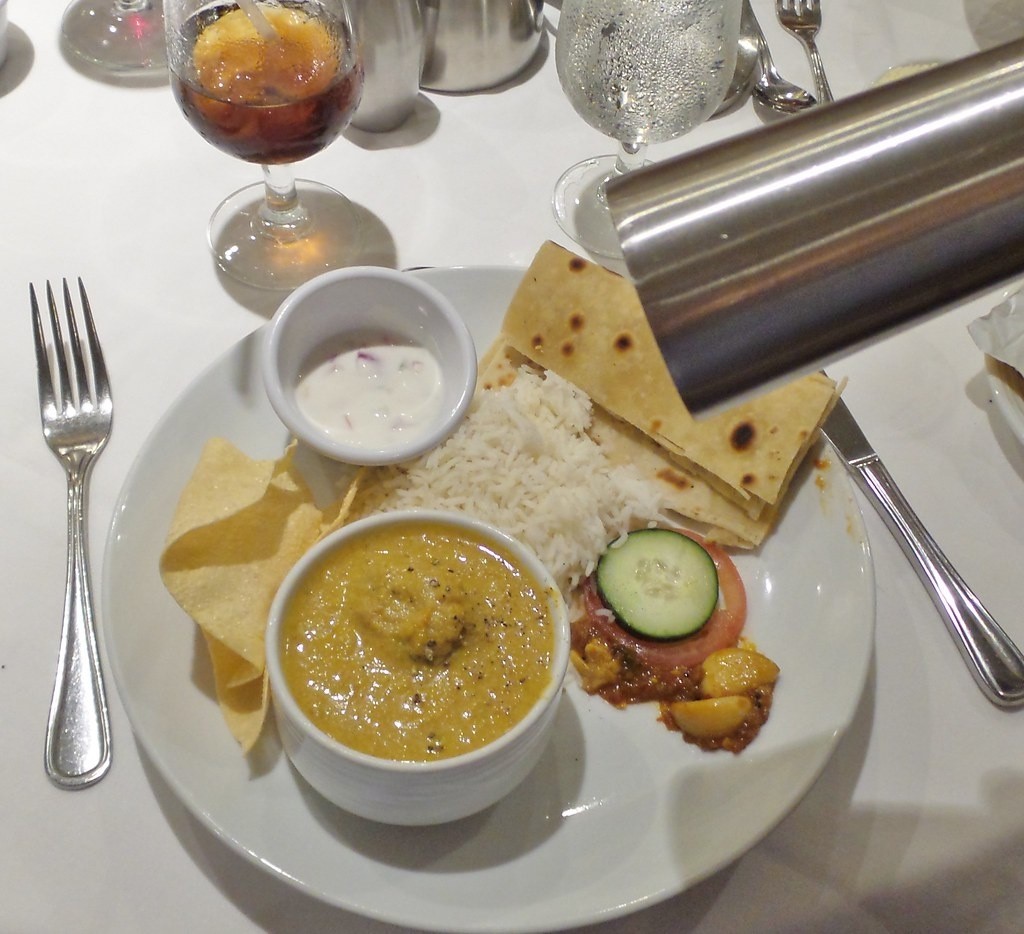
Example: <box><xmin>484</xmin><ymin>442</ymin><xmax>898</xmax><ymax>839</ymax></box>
<box><xmin>476</xmin><ymin>243</ymin><xmax>836</xmax><ymax>548</ymax></box>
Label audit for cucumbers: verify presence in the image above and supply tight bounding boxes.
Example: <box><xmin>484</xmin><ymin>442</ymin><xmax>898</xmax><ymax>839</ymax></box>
<box><xmin>598</xmin><ymin>530</ymin><xmax>719</xmax><ymax>639</ymax></box>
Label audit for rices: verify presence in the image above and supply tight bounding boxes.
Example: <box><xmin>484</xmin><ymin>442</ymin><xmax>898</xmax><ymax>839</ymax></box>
<box><xmin>362</xmin><ymin>365</ymin><xmax>657</xmax><ymax>588</ymax></box>
